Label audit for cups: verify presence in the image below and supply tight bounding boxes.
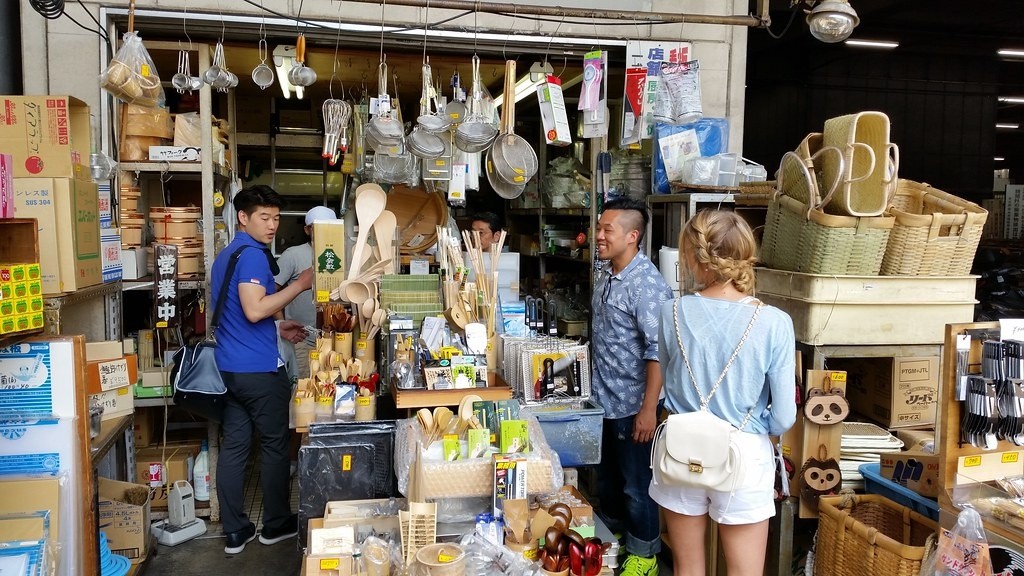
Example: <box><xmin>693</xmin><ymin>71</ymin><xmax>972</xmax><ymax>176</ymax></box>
<box><xmin>570</xmin><ymin>568</ymin><xmax>601</xmax><ymax>576</ymax></box>
<box><xmin>505</xmin><ymin>532</ymin><xmax>539</xmax><ymax>563</ymax></box>
<box><xmin>417</xmin><ymin>544</ymin><xmax>465</xmax><ymax>575</ymax></box>
<box><xmin>539</xmin><ymin>567</ymin><xmax>569</xmax><ymax>575</ymax></box>
<box><xmin>294</xmin><ymin>332</ymin><xmax>375</xmax><ymax>427</ymax></box>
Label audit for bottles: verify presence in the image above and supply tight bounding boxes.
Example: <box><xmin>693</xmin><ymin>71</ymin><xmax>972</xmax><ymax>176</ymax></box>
<box><xmin>193</xmin><ymin>439</ymin><xmax>210</xmax><ymax>502</ymax></box>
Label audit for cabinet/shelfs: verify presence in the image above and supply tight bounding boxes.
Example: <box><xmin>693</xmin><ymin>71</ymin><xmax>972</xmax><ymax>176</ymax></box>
<box><xmin>504</xmin><ymin>97</ymin><xmax>674</xmax><ymax>351</ymax></box>
<box><xmin>41</xmin><ymin>278</ymin><xmax>158</xmax><ymax>576</ymax></box>
<box><xmin>115</xmin><ymin>39</ymin><xmax>238</xmax><ymax>522</ymax></box>
<box><xmin>764</xmin><ymin>323</ymin><xmax>1024</xmax><ymax>576</ymax></box>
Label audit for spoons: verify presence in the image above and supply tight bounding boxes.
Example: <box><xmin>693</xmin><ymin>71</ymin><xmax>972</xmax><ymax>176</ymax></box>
<box><xmin>538</xmin><ymin>502</ymin><xmax>611</xmax><ymax>575</ymax></box>
<box><xmin>965</xmin><ymin>378</ymin><xmax>1024</xmax><ymax>451</ymax></box>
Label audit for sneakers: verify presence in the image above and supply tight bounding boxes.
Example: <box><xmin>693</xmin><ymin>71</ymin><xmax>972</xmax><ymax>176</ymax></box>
<box><xmin>619</xmin><ymin>553</ymin><xmax>659</xmax><ymax>576</ymax></box>
<box><xmin>225</xmin><ymin>522</ymin><xmax>257</xmax><ymax>553</ymax></box>
<box><xmin>612</xmin><ymin>534</ymin><xmax>627</xmax><ymax>554</ymax></box>
<box><xmin>259</xmin><ymin>512</ymin><xmax>300</xmax><ymax>545</ymax></box>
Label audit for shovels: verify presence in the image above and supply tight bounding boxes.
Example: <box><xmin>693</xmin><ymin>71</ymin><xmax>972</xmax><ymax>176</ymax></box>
<box><xmin>417</xmin><ymin>407</ymin><xmax>458</xmax><ymax>450</ymax></box>
<box><xmin>450</xmin><ymin>304</ymin><xmax>468</xmax><ymax>330</ymax></box>
<box><xmin>455</xmin><ymin>395</ymin><xmax>484</xmax><ymax>438</ymax></box>
<box><xmin>528</xmin><ymin>509</ymin><xmax>557</xmax><ymax>544</ymax></box>
<box><xmin>463</xmin><ymin>321</ymin><xmax>488</xmax><ymax>353</ymax></box>
<box><xmin>504</xmin><ymin>499</ymin><xmax>529</xmax><ymax>545</ymax></box>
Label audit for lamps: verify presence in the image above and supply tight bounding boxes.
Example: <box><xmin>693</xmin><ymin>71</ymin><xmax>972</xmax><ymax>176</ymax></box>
<box><xmin>271</xmin><ymin>43</ymin><xmax>305</xmax><ymax>100</ymax></box>
<box><xmin>803</xmin><ymin>0</ymin><xmax>859</xmax><ymax>43</ymax></box>
<box><xmin>489</xmin><ymin>62</ymin><xmax>554</xmax><ymax>109</ymax></box>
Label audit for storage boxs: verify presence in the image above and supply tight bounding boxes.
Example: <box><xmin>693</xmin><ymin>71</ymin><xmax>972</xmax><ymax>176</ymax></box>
<box><xmin>752</xmin><ymin>267</ymin><xmax>981</xmax><ymax>498</ymax></box>
<box><xmin>305</xmin><ymin>399</ymin><xmax>605</xmax><ymax>576</ymax></box>
<box><xmin>0</xmin><ymin>94</ymin><xmax>201</xmax><ymax>566</ymax></box>
<box><xmin>537</xmin><ymin>75</ymin><xmax>572</xmax><ymax>147</ymax></box>
<box><xmin>311</xmin><ymin>220</ymin><xmax>348</xmax><ymax>304</ymax></box>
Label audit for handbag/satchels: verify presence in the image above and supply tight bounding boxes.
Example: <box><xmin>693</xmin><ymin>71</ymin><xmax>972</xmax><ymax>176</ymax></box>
<box><xmin>170</xmin><ymin>340</ymin><xmax>227</xmax><ymax>416</ymax></box>
<box><xmin>821</xmin><ymin>110</ymin><xmax>899</xmax><ymax>216</ymax></box>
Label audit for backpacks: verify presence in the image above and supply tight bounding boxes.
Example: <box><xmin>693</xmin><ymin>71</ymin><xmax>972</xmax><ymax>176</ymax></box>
<box><xmin>649</xmin><ymin>294</ymin><xmax>763</xmax><ymax>493</ymax></box>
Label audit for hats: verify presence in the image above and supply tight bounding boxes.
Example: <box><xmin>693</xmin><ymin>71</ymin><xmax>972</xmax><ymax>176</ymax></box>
<box><xmin>305</xmin><ymin>206</ymin><xmax>337</xmax><ymax>225</ymax></box>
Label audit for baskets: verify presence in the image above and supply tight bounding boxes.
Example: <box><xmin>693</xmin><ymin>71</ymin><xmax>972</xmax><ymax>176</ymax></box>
<box><xmin>814</xmin><ymin>490</ymin><xmax>941</xmax><ymax>576</ymax></box>
<box><xmin>756</xmin><ymin>130</ymin><xmax>989</xmax><ymax>278</ymax></box>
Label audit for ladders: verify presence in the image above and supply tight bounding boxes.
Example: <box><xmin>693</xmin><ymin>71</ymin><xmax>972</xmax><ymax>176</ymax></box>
<box><xmin>270</xmin><ymin>97</ymin><xmax>328</xmax><ymax>258</ymax></box>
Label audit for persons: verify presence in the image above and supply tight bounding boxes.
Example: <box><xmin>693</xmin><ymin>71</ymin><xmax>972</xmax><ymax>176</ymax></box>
<box><xmin>271</xmin><ymin>205</ymin><xmax>339</xmax><ymax>382</ymax></box>
<box><xmin>586</xmin><ymin>195</ymin><xmax>674</xmax><ymax>575</ymax></box>
<box><xmin>211</xmin><ymin>183</ymin><xmax>315</xmax><ymax>555</ymax></box>
<box><xmin>649</xmin><ymin>207</ymin><xmax>798</xmax><ymax>576</ymax></box>
<box><xmin>471</xmin><ymin>211</ymin><xmax>505</xmax><ymax>254</ymax></box>
